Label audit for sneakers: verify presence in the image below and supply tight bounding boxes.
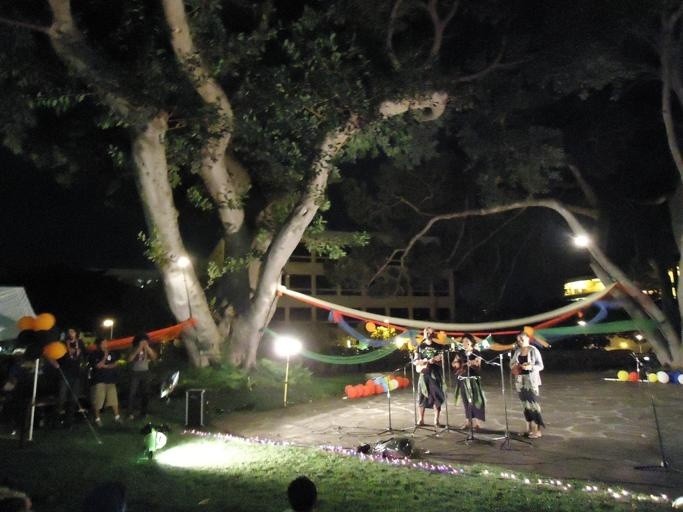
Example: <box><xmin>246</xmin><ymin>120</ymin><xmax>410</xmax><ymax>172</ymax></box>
<box><xmin>415</xmin><ymin>420</ymin><xmax>483</xmax><ymax>433</ymax></box>
<box><xmin>518</xmin><ymin>431</ymin><xmax>542</xmax><ymax>439</ymax></box>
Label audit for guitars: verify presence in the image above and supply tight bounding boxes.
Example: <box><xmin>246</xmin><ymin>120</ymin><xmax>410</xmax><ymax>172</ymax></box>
<box><xmin>452</xmin><ymin>357</ymin><xmax>481</xmax><ymax>377</ymax></box>
<box><xmin>416</xmin><ymin>354</ymin><xmax>442</xmax><ymax>373</ymax></box>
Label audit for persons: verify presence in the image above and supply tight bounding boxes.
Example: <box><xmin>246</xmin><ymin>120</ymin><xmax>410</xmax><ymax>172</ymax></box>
<box><xmin>507</xmin><ymin>331</ymin><xmax>545</xmax><ymax>439</ymax></box>
<box><xmin>450</xmin><ymin>332</ymin><xmax>486</xmax><ymax>433</ymax></box>
<box><xmin>411</xmin><ymin>325</ymin><xmax>460</xmax><ymax>431</ymax></box>
<box><xmin>285</xmin><ymin>476</ymin><xmax>320</xmax><ymax>512</ymax></box>
<box><xmin>0</xmin><ymin>486</ymin><xmax>35</xmax><ymax>511</ymax></box>
<box><xmin>55</xmin><ymin>325</ymin><xmax>87</xmax><ymax>420</ymax></box>
<box><xmin>88</xmin><ymin>333</ymin><xmax>125</xmax><ymax>429</ymax></box>
<box><xmin>127</xmin><ymin>332</ymin><xmax>158</xmax><ymax>422</ymax></box>
<box><xmin>81</xmin><ymin>481</ymin><xmax>126</xmax><ymax>511</ymax></box>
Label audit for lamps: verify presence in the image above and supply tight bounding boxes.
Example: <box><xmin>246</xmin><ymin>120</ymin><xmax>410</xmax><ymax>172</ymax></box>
<box><xmin>144</xmin><ymin>428</ymin><xmax>167</xmax><ymax>463</ymax></box>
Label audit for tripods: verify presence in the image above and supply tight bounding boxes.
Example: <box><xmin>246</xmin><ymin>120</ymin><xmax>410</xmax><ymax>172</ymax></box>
<box><xmin>377</xmin><ymin>356</ymin><xmax>532</xmax><ymax>450</ymax></box>
<box><xmin>633</xmin><ymin>395</ymin><xmax>683</xmax><ymax>475</ymax></box>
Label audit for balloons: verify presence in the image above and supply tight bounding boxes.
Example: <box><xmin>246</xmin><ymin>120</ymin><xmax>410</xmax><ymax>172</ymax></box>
<box><xmin>677</xmin><ymin>373</ymin><xmax>683</xmax><ymax>384</ymax></box>
<box><xmin>342</xmin><ymin>372</ymin><xmax>409</xmax><ymax>401</ymax></box>
<box><xmin>17</xmin><ymin>329</ymin><xmax>34</xmax><ymax>345</ymax></box>
<box><xmin>37</xmin><ymin>329</ymin><xmax>57</xmax><ymax>346</ymax></box>
<box><xmin>656</xmin><ymin>370</ymin><xmax>668</xmax><ymax>384</ymax></box>
<box><xmin>16</xmin><ymin>315</ymin><xmax>35</xmax><ymax>331</ymax></box>
<box><xmin>25</xmin><ymin>342</ymin><xmax>41</xmax><ymax>360</ymax></box>
<box><xmin>617</xmin><ymin>370</ymin><xmax>628</xmax><ymax>382</ymax></box>
<box><xmin>42</xmin><ymin>340</ymin><xmax>67</xmax><ymax>360</ymax></box>
<box><xmin>647</xmin><ymin>372</ymin><xmax>657</xmax><ymax>382</ymax></box>
<box><xmin>628</xmin><ymin>371</ymin><xmax>638</xmax><ymax>382</ymax></box>
<box><xmin>33</xmin><ymin>313</ymin><xmax>56</xmax><ymax>332</ymax></box>
<box><xmin>669</xmin><ymin>369</ymin><xmax>680</xmax><ymax>384</ymax></box>
